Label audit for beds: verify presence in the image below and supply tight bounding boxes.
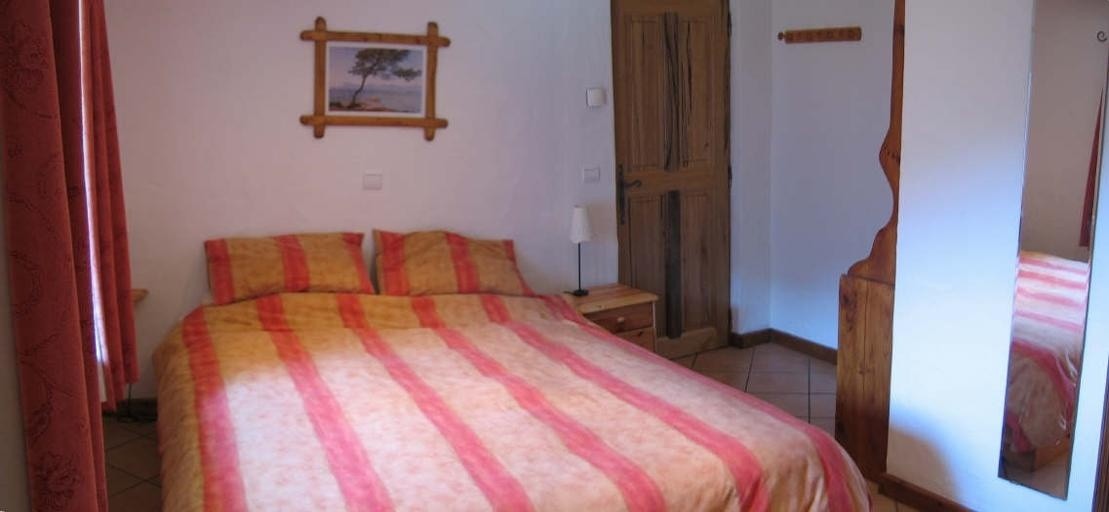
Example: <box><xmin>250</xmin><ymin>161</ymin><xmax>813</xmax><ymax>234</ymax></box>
<box><xmin>1000</xmin><ymin>248</ymin><xmax>1091</xmax><ymax>472</ymax></box>
<box><xmin>171</xmin><ymin>291</ymin><xmax>861</xmax><ymax>510</ymax></box>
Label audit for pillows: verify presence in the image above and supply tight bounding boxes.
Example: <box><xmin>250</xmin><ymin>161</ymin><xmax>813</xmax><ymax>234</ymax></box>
<box><xmin>373</xmin><ymin>230</ymin><xmax>531</xmax><ymax>297</ymax></box>
<box><xmin>203</xmin><ymin>232</ymin><xmax>376</xmax><ymax>305</ymax></box>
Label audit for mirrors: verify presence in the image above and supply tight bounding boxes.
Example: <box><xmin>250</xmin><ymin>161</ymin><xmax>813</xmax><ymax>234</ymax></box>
<box><xmin>997</xmin><ymin>0</ymin><xmax>1108</xmax><ymax>503</ymax></box>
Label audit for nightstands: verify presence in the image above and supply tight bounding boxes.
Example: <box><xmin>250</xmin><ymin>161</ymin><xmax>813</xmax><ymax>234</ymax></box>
<box><xmin>558</xmin><ymin>282</ymin><xmax>660</xmax><ymax>356</ymax></box>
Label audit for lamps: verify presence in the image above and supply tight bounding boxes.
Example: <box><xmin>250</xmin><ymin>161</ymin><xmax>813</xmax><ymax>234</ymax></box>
<box><xmin>569</xmin><ymin>206</ymin><xmax>595</xmax><ymax>298</ymax></box>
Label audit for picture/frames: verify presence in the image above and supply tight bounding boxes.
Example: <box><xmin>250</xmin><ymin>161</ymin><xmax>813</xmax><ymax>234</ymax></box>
<box><xmin>298</xmin><ymin>16</ymin><xmax>451</xmax><ymax>141</ymax></box>
